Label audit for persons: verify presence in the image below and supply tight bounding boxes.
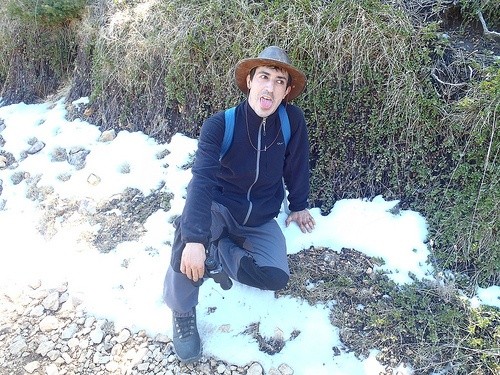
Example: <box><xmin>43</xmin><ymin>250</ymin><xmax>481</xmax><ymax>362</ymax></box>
<box><xmin>163</xmin><ymin>46</ymin><xmax>316</xmax><ymax>363</ymax></box>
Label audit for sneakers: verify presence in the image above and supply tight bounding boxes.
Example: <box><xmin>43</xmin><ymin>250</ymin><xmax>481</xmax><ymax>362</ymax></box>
<box><xmin>172</xmin><ymin>308</ymin><xmax>201</xmax><ymax>362</ymax></box>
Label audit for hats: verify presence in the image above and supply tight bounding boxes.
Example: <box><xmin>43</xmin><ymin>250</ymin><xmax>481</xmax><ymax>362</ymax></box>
<box><xmin>235</xmin><ymin>47</ymin><xmax>308</xmax><ymax>102</ymax></box>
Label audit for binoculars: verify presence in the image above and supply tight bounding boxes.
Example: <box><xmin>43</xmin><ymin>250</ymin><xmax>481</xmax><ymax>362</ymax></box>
<box><xmin>190</xmin><ymin>256</ymin><xmax>233</xmax><ymax>290</ymax></box>
